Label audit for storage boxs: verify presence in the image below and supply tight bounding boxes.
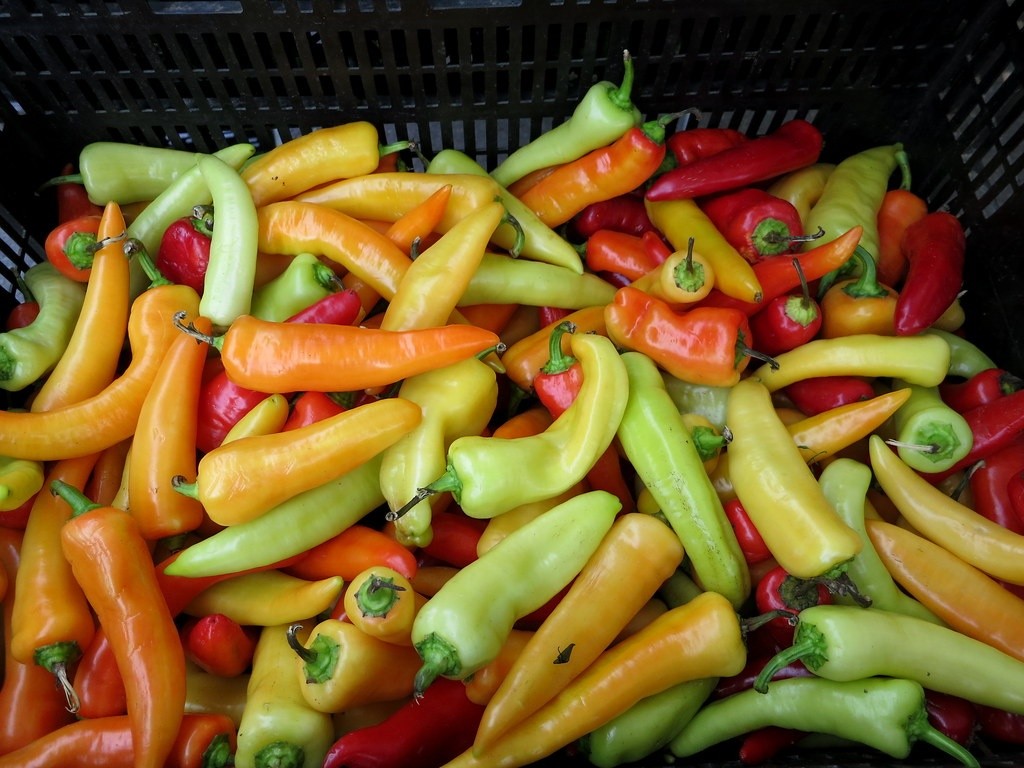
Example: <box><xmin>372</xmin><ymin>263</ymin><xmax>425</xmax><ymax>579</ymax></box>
<box><xmin>0</xmin><ymin>1</ymin><xmax>1023</xmax><ymax>768</ymax></box>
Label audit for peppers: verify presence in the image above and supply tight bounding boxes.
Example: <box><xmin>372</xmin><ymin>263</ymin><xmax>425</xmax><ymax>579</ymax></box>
<box><xmin>0</xmin><ymin>52</ymin><xmax>1024</xmax><ymax>768</ymax></box>
